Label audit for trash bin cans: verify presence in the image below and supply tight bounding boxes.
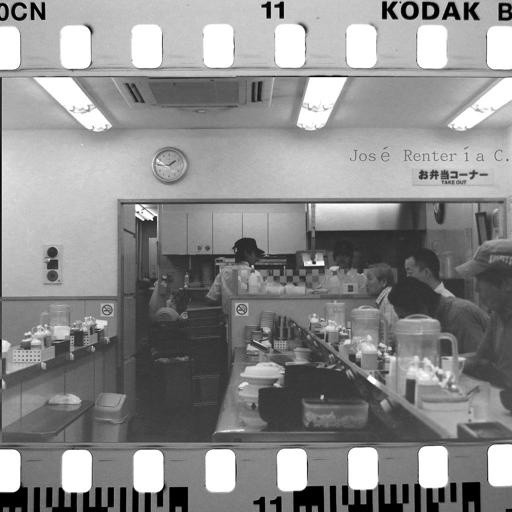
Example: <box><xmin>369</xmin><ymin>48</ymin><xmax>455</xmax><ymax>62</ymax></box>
<box><xmin>91</xmin><ymin>392</ymin><xmax>129</xmax><ymax>443</ymax></box>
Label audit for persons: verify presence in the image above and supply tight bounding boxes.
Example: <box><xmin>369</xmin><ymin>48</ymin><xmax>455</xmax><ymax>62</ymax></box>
<box><xmin>453</xmin><ymin>238</ymin><xmax>511</xmax><ymax>386</ymax></box>
<box><xmin>364</xmin><ymin>262</ymin><xmax>400</xmax><ymax>328</ymax></box>
<box><xmin>384</xmin><ymin>276</ymin><xmax>489</xmax><ymax>354</ymax></box>
<box><xmin>204</xmin><ymin>238</ymin><xmax>267</xmax><ymax>345</ymax></box>
<box><xmin>403</xmin><ymin>248</ymin><xmax>456</xmax><ymax>298</ymax></box>
<box><xmin>328</xmin><ymin>238</ymin><xmax>356</xmax><ymax>272</ymax></box>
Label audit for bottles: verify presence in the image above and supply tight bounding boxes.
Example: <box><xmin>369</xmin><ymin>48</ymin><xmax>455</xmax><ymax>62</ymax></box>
<box><xmin>21</xmin><ymin>325</ymin><xmax>52</xmax><ymax>350</ymax></box>
<box><xmin>73</xmin><ymin>316</ymin><xmax>97</xmax><ymax>336</ymax></box>
<box><xmin>184</xmin><ymin>270</ymin><xmax>189</xmax><ymax>287</ymax></box>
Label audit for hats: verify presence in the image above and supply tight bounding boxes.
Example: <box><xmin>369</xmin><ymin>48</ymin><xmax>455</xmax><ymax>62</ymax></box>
<box><xmin>455</xmin><ymin>239</ymin><xmax>512</xmax><ymax>278</ymax></box>
<box><xmin>232</xmin><ymin>238</ymin><xmax>265</xmax><ymax>256</ymax></box>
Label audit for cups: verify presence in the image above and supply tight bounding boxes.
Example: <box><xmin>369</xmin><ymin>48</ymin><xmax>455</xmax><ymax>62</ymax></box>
<box><xmin>323</xmin><ymin>301</ymin><xmax>347</xmax><ymax>331</ymax></box>
<box><xmin>441</xmin><ymin>356</ymin><xmax>465</xmax><ymax>384</ymax></box>
<box><xmin>350</xmin><ymin>305</ymin><xmax>386</xmax><ymax>361</ymax></box>
<box><xmin>392</xmin><ymin>314</ymin><xmax>458</xmax><ymax>392</ymax></box>
<box><xmin>40</xmin><ymin>303</ymin><xmax>72</xmax><ymax>344</ymax></box>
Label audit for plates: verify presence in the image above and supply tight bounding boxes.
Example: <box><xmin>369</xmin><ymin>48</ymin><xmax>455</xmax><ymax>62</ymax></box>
<box><xmin>240</xmin><ymin>372</ymin><xmax>279</xmax><ymax>386</ymax></box>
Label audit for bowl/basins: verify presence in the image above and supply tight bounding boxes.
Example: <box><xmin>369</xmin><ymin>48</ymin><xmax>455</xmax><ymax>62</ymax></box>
<box><xmin>293</xmin><ymin>347</ymin><xmax>313</xmax><ymax>360</ymax></box>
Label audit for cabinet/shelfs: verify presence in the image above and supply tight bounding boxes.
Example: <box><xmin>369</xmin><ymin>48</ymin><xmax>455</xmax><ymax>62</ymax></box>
<box><xmin>157</xmin><ymin>203</ymin><xmax>308</xmax><ymax>256</ymax></box>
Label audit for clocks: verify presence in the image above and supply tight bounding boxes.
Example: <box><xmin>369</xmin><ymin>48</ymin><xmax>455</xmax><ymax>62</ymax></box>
<box><xmin>150</xmin><ymin>146</ymin><xmax>190</xmax><ymax>186</ymax></box>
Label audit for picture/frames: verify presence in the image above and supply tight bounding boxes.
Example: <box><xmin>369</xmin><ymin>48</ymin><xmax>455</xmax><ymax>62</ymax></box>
<box><xmin>474</xmin><ymin>210</ymin><xmax>489</xmax><ymax>247</ymax></box>
